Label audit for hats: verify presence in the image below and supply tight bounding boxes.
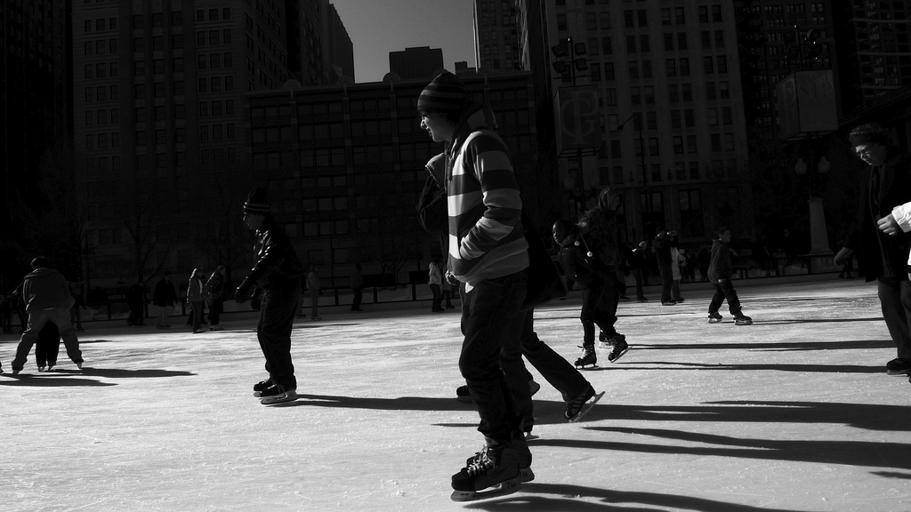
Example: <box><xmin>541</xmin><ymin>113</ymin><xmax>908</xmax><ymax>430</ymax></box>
<box><xmin>243</xmin><ymin>192</ymin><xmax>271</xmax><ymax>215</ymax></box>
<box><xmin>850</xmin><ymin>123</ymin><xmax>886</xmax><ymax>146</ymax></box>
<box><xmin>416</xmin><ymin>69</ymin><xmax>477</xmax><ymax>113</ymax></box>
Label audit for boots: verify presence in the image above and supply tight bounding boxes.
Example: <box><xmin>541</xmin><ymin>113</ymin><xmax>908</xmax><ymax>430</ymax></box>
<box><xmin>607</xmin><ymin>332</ymin><xmax>632</xmax><ymax>363</ymax></box>
<box><xmin>451</xmin><ymin>437</ymin><xmax>536</xmax><ymax>501</ymax></box>
<box><xmin>574</xmin><ymin>342</ymin><xmax>600</xmax><ymax>370</ymax></box>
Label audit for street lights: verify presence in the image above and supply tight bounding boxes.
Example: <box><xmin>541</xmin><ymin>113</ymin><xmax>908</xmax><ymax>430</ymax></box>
<box><xmin>794</xmin><ymin>156</ymin><xmax>835</xmax><ymax>266</ymax></box>
<box><xmin>550</xmin><ymin>36</ymin><xmax>592</xmax><ymax>211</ymax></box>
<box><xmin>617</xmin><ymin>110</ymin><xmax>652</xmax><ymax>238</ymax></box>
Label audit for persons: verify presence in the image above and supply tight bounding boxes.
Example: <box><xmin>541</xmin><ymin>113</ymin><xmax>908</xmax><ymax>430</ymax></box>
<box><xmin>232</xmin><ymin>182</ymin><xmax>301</xmax><ymax>397</ymax></box>
<box><xmin>552</xmin><ymin>186</ymin><xmax>628</xmax><ymax>366</ymax></box>
<box><xmin>535</xmin><ymin>230</ymin><xmax>683</xmax><ymax>303</ymax></box>
<box><xmin>3</xmin><ymin>255</ymin><xmax>82</xmax><ymax>377</ymax></box>
<box><xmin>832</xmin><ymin>121</ymin><xmax>910</xmax><ymax>382</ymax></box>
<box><xmin>417</xmin><ymin>151</ymin><xmax>595</xmax><ymax>420</ymax></box>
<box><xmin>35</xmin><ymin>332</ymin><xmax>59</xmax><ymax>366</ymax></box>
<box><xmin>305</xmin><ymin>265</ymin><xmax>320</xmax><ymax>319</ymax></box>
<box><xmin>876</xmin><ymin>200</ymin><xmax>910</xmax><ymax>285</ymax></box>
<box><xmin>126</xmin><ymin>265</ymin><xmax>228</xmax><ymax>333</ymax></box>
<box><xmin>707</xmin><ymin>226</ymin><xmax>752</xmax><ymax>322</ymax></box>
<box><xmin>414</xmin><ymin>70</ymin><xmax>532</xmax><ymax>491</ymax></box>
<box><xmin>348</xmin><ymin>264</ymin><xmax>364</xmax><ymax>311</ymax></box>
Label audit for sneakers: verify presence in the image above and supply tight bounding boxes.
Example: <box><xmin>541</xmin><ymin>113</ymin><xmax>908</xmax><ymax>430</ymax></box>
<box><xmin>523</xmin><ymin>412</ymin><xmax>541</xmax><ymax>442</ymax></box>
<box><xmin>73</xmin><ymin>355</ymin><xmax>84</xmax><ymax>368</ymax></box>
<box><xmin>887</xmin><ymin>357</ymin><xmax>911</xmax><ymax>375</ymax></box>
<box><xmin>37</xmin><ymin>359</ymin><xmax>57</xmax><ymax>371</ymax></box>
<box><xmin>253</xmin><ymin>376</ymin><xmax>300</xmax><ymax>405</ymax></box>
<box><xmin>661</xmin><ymin>296</ymin><xmax>685</xmax><ymax>306</ymax></box>
<box><xmin>564</xmin><ymin>379</ymin><xmax>607</xmax><ymax>424</ymax></box>
<box><xmin>12</xmin><ymin>363</ymin><xmax>24</xmax><ymax>375</ymax></box>
<box><xmin>457</xmin><ymin>384</ymin><xmax>475</xmax><ymax>403</ymax></box>
<box><xmin>707</xmin><ymin>312</ymin><xmax>724</xmax><ymax>323</ymax></box>
<box><xmin>733</xmin><ymin>312</ymin><xmax>754</xmax><ymax>326</ymax></box>
<box><xmin>599</xmin><ymin>327</ymin><xmax>626</xmax><ymax>347</ymax></box>
